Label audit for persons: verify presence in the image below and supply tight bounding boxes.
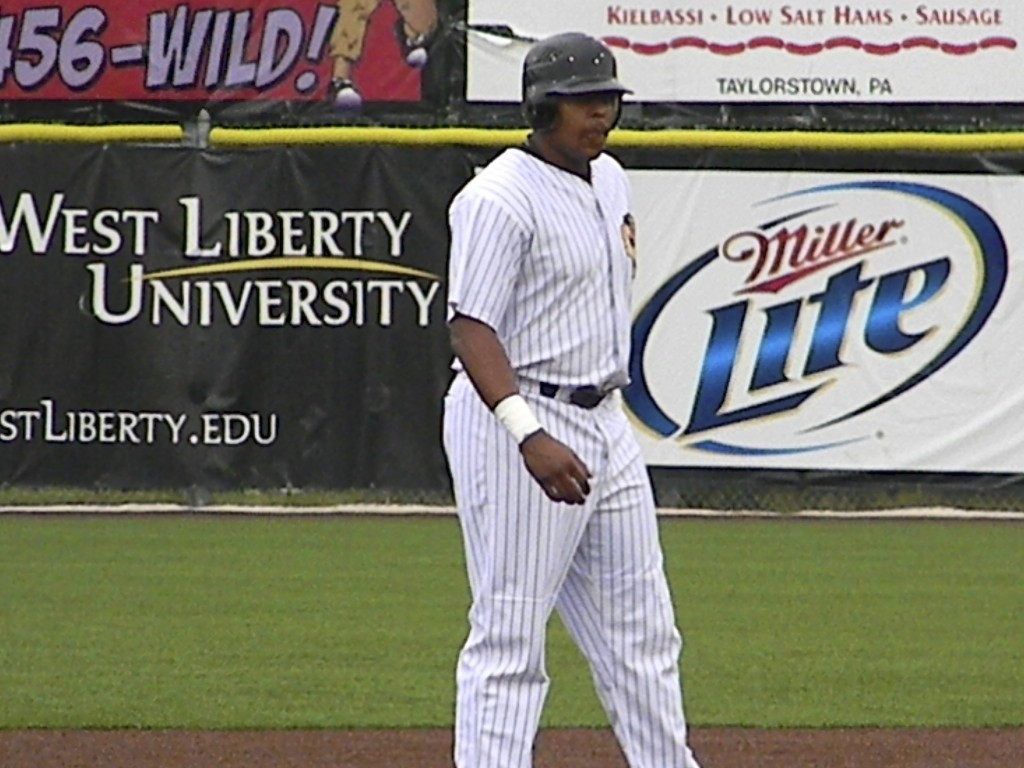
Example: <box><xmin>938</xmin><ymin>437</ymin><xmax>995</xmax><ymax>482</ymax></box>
<box><xmin>440</xmin><ymin>29</ymin><xmax>701</xmax><ymax>768</ymax></box>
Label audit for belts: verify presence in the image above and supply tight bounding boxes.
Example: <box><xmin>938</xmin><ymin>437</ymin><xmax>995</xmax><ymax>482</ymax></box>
<box><xmin>539</xmin><ymin>382</ymin><xmax>604</xmax><ymax>410</ymax></box>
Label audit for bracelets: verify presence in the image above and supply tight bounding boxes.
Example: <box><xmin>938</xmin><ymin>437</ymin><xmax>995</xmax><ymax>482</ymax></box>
<box><xmin>490</xmin><ymin>391</ymin><xmax>543</xmax><ymax>451</ymax></box>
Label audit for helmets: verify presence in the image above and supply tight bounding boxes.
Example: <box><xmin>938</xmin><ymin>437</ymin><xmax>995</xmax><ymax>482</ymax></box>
<box><xmin>522</xmin><ymin>32</ymin><xmax>633</xmax><ymax>139</ymax></box>
